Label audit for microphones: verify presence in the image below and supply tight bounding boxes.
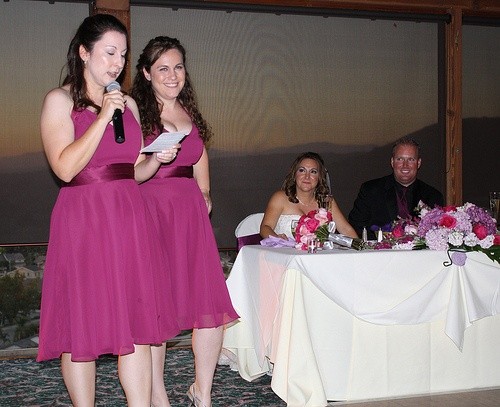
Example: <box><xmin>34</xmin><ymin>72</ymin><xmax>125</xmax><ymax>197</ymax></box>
<box><xmin>106</xmin><ymin>81</ymin><xmax>125</xmax><ymax>143</ymax></box>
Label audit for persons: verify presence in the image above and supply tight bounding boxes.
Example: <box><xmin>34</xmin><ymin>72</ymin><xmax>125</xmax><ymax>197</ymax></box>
<box><xmin>127</xmin><ymin>36</ymin><xmax>241</xmax><ymax>407</ymax></box>
<box><xmin>346</xmin><ymin>138</ymin><xmax>443</xmax><ymax>239</ymax></box>
<box><xmin>261</xmin><ymin>151</ymin><xmax>360</xmax><ymax>240</ymax></box>
<box><xmin>35</xmin><ymin>14</ymin><xmax>182</xmax><ymax>406</ymax></box>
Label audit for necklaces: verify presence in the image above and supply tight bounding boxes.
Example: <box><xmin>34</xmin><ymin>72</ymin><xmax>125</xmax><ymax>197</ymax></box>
<box><xmin>294</xmin><ymin>191</ymin><xmax>316</xmax><ymax>208</ymax></box>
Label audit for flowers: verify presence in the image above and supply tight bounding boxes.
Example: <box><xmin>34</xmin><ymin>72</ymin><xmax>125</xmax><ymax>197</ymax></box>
<box><xmin>366</xmin><ymin>200</ymin><xmax>500</xmax><ymax>266</ymax></box>
<box><xmin>295</xmin><ymin>207</ymin><xmax>366</xmax><ymax>251</ymax></box>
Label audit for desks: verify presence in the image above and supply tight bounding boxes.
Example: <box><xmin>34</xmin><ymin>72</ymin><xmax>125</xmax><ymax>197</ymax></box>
<box><xmin>216</xmin><ymin>244</ymin><xmax>500</xmax><ymax>407</ymax></box>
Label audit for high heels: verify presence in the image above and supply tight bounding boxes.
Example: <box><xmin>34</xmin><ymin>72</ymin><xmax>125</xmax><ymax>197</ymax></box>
<box><xmin>185</xmin><ymin>383</ymin><xmax>213</xmax><ymax>407</ymax></box>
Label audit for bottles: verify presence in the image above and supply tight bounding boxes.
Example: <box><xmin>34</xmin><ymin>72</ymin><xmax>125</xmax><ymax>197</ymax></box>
<box><xmin>377</xmin><ymin>228</ymin><xmax>383</xmax><ymax>242</ymax></box>
<box><xmin>362</xmin><ymin>227</ymin><xmax>367</xmax><ymax>242</ymax></box>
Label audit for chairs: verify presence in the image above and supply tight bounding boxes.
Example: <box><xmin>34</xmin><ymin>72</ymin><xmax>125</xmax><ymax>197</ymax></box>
<box><xmin>234</xmin><ymin>213</ymin><xmax>265</xmax><ymax>255</ymax></box>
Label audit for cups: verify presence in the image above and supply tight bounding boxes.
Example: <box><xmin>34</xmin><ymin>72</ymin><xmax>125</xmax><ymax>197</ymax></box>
<box><xmin>307</xmin><ymin>239</ymin><xmax>317</xmax><ymax>255</ymax></box>
<box><xmin>291</xmin><ymin>220</ymin><xmax>299</xmax><ymax>239</ymax></box>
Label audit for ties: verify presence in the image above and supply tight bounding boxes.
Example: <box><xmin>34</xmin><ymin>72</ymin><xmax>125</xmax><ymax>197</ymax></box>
<box><xmin>400</xmin><ymin>188</ymin><xmax>408</xmax><ymax>206</ymax></box>
